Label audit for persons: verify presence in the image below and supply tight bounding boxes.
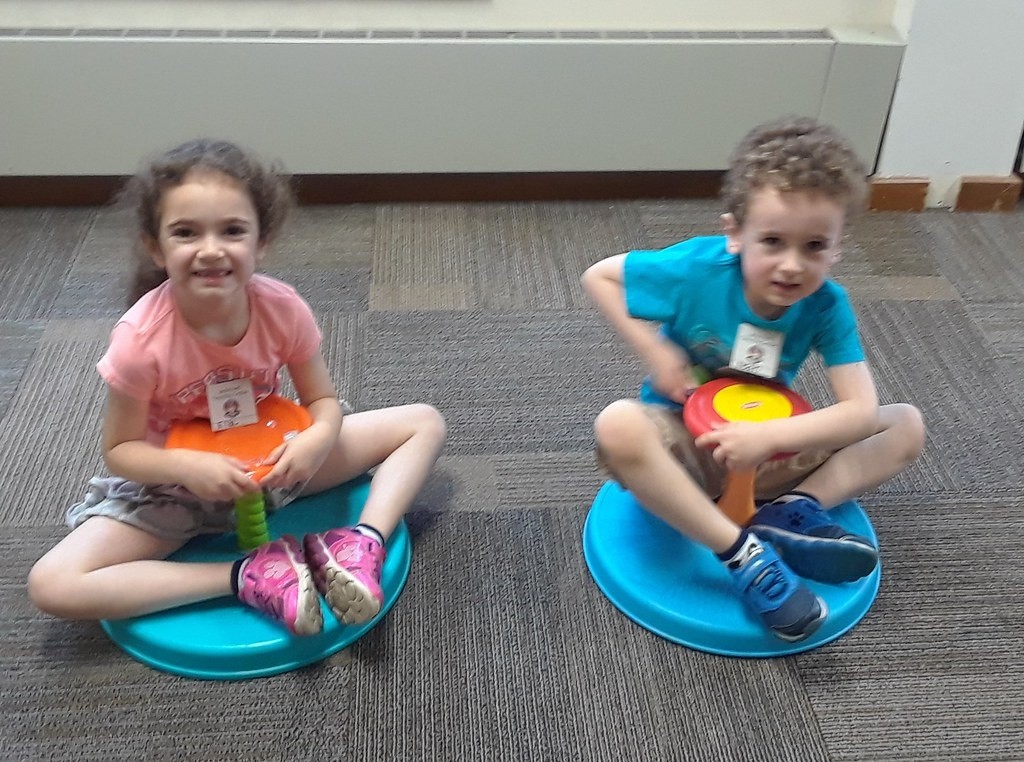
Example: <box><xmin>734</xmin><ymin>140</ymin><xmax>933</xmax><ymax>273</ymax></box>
<box><xmin>26</xmin><ymin>137</ymin><xmax>447</xmax><ymax>637</ymax></box>
<box><xmin>579</xmin><ymin>115</ymin><xmax>925</xmax><ymax>643</ymax></box>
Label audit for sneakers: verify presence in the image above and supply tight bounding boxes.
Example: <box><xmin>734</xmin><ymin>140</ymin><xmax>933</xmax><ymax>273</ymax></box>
<box><xmin>237</xmin><ymin>534</ymin><xmax>323</xmax><ymax>637</ymax></box>
<box><xmin>745</xmin><ymin>490</ymin><xmax>880</xmax><ymax>586</ymax></box>
<box><xmin>302</xmin><ymin>526</ymin><xmax>385</xmax><ymax>630</ymax></box>
<box><xmin>721</xmin><ymin>533</ymin><xmax>829</xmax><ymax>645</ymax></box>
